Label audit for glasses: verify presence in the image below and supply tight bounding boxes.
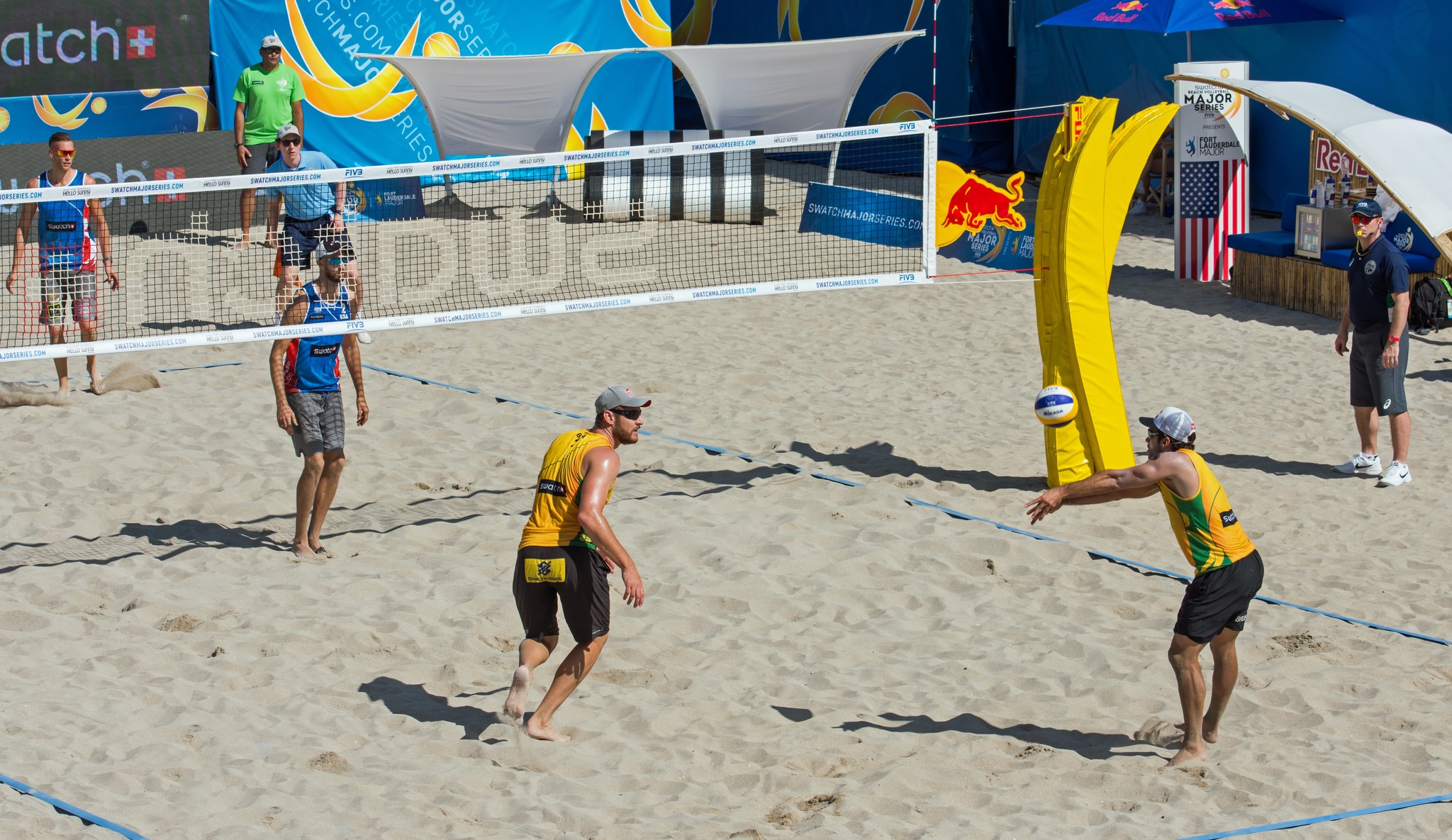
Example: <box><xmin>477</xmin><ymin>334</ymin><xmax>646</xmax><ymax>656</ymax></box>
<box><xmin>1351</xmin><ymin>216</ymin><xmax>1378</xmax><ymax>224</ymax></box>
<box><xmin>611</xmin><ymin>410</ymin><xmax>642</xmax><ymax>420</ymax></box>
<box><xmin>280</xmin><ymin>139</ymin><xmax>300</xmax><ymax>146</ymax></box>
<box><xmin>1148</xmin><ymin>429</ymin><xmax>1172</xmax><ymax>441</ymax></box>
<box><xmin>265</xmin><ymin>48</ymin><xmax>281</xmax><ymax>54</ymax></box>
<box><xmin>51</xmin><ymin>148</ymin><xmax>77</xmax><ymax>157</ymax></box>
<box><xmin>321</xmin><ymin>257</ymin><xmax>350</xmax><ymax>266</ymax></box>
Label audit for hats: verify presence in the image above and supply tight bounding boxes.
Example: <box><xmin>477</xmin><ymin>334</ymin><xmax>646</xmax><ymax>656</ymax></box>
<box><xmin>595</xmin><ymin>386</ymin><xmax>651</xmax><ymax>414</ymax></box>
<box><xmin>1350</xmin><ymin>198</ymin><xmax>1382</xmax><ymax>217</ymax></box>
<box><xmin>315</xmin><ymin>239</ymin><xmax>354</xmax><ymax>262</ymax></box>
<box><xmin>277</xmin><ymin>124</ymin><xmax>300</xmax><ymax>138</ymax></box>
<box><xmin>1139</xmin><ymin>406</ymin><xmax>1196</xmax><ymax>443</ymax></box>
<box><xmin>261</xmin><ymin>35</ymin><xmax>282</xmax><ymax>48</ymax></box>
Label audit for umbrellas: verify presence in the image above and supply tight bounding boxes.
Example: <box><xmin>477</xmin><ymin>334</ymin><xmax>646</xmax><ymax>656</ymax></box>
<box><xmin>1032</xmin><ymin>0</ymin><xmax>1347</xmax><ymax>61</ymax></box>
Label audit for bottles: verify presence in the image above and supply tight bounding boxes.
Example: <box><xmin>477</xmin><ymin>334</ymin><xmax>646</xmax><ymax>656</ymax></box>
<box><xmin>1340</xmin><ymin>157</ymin><xmax>1350</xmax><ymax>179</ymax></box>
<box><xmin>1368</xmin><ymin>174</ymin><xmax>1374</xmax><ymax>187</ymax></box>
<box><xmin>1313</xmin><ymin>180</ymin><xmax>1320</xmax><ymax>189</ymax></box>
<box><xmin>1326</xmin><ymin>176</ymin><xmax>1336</xmax><ymax>208</ymax></box>
<box><xmin>1316</xmin><ymin>182</ymin><xmax>1325</xmax><ymax>208</ymax></box>
<box><xmin>1341</xmin><ymin>174</ymin><xmax>1351</xmax><ymax>205</ymax></box>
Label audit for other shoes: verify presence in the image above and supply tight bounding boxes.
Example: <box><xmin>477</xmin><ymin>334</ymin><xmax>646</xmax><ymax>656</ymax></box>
<box><xmin>356</xmin><ymin>332</ymin><xmax>372</xmax><ymax>343</ymax></box>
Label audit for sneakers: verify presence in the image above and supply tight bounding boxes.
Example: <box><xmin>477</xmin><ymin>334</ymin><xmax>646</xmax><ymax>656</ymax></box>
<box><xmin>1378</xmin><ymin>460</ymin><xmax>1412</xmax><ymax>487</ymax></box>
<box><xmin>1333</xmin><ymin>451</ymin><xmax>1383</xmax><ymax>475</ymax></box>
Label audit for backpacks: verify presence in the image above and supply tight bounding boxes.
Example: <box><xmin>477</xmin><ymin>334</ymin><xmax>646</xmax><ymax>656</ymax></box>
<box><xmin>1412</xmin><ymin>275</ymin><xmax>1452</xmax><ymax>329</ymax></box>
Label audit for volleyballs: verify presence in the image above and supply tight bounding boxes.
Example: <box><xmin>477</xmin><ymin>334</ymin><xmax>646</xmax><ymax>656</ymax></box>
<box><xmin>1034</xmin><ymin>384</ymin><xmax>1078</xmax><ymax>429</ymax></box>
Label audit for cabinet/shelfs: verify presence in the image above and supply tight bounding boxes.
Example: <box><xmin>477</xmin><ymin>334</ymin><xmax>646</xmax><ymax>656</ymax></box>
<box><xmin>1294</xmin><ymin>204</ymin><xmax>1358</xmax><ymax>259</ymax></box>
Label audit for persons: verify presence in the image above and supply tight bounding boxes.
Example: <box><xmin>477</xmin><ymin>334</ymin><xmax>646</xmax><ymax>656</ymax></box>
<box><xmin>231</xmin><ymin>35</ymin><xmax>308</xmax><ymax>252</ymax></box>
<box><xmin>267</xmin><ymin>124</ymin><xmax>372</xmax><ymax>343</ymax></box>
<box><xmin>504</xmin><ymin>387</ymin><xmax>651</xmax><ymax>744</ymax></box>
<box><xmin>1331</xmin><ymin>198</ymin><xmax>1410</xmax><ymax>486</ymax></box>
<box><xmin>4</xmin><ymin>132</ymin><xmax>120</xmax><ymax>401</ymax></box>
<box><xmin>268</xmin><ymin>236</ymin><xmax>370</xmax><ymax>563</ymax></box>
<box><xmin>1024</xmin><ymin>406</ymin><xmax>1264</xmax><ymax>775</ymax></box>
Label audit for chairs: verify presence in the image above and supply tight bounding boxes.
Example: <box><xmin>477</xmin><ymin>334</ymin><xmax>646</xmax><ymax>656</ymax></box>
<box><xmin>1227</xmin><ymin>193</ymin><xmax>1329</xmax><ymax>259</ymax></box>
<box><xmin>1130</xmin><ymin>146</ymin><xmax>1174</xmax><ymax>216</ymax></box>
<box><xmin>1320</xmin><ymin>208</ymin><xmax>1441</xmax><ymax>275</ymax></box>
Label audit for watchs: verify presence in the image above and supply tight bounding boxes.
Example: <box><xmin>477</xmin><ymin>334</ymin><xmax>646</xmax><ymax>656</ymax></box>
<box><xmin>234</xmin><ymin>143</ymin><xmax>243</xmax><ymax>149</ymax></box>
<box><xmin>1388</xmin><ymin>335</ymin><xmax>1400</xmax><ymax>342</ymax></box>
<box><xmin>102</xmin><ymin>256</ymin><xmax>113</xmax><ymax>265</ymax></box>
<box><xmin>335</xmin><ymin>209</ymin><xmax>344</xmax><ymax>216</ymax></box>
